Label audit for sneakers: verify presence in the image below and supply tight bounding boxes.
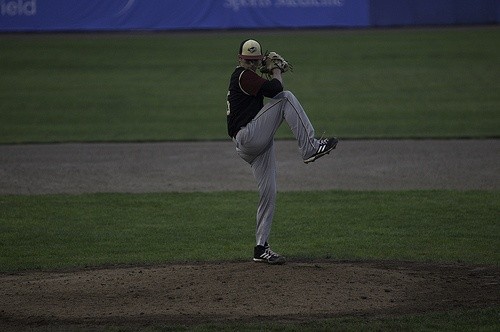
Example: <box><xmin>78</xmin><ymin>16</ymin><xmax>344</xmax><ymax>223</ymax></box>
<box><xmin>301</xmin><ymin>135</ymin><xmax>338</xmax><ymax>165</ymax></box>
<box><xmin>253</xmin><ymin>243</ymin><xmax>285</xmax><ymax>265</ymax></box>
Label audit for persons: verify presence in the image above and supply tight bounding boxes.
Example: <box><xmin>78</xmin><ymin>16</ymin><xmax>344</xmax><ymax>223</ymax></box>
<box><xmin>227</xmin><ymin>38</ymin><xmax>338</xmax><ymax>264</ymax></box>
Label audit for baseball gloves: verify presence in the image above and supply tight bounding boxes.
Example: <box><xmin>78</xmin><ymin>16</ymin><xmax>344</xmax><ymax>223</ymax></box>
<box><xmin>259</xmin><ymin>49</ymin><xmax>293</xmax><ymax>80</ymax></box>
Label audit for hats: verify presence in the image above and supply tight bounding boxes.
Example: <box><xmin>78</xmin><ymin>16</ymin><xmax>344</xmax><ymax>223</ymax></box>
<box><xmin>238</xmin><ymin>38</ymin><xmax>263</xmax><ymax>60</ymax></box>
